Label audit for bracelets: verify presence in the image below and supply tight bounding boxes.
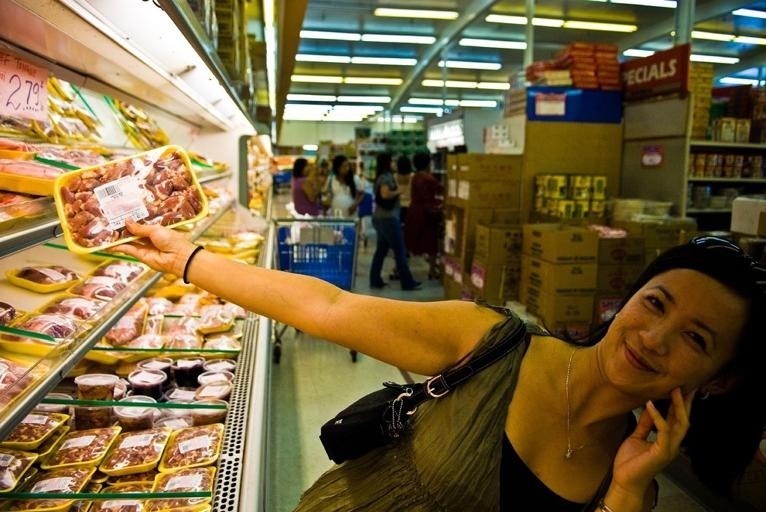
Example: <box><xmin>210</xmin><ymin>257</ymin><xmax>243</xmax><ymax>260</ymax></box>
<box><xmin>182</xmin><ymin>246</ymin><xmax>204</xmax><ymax>284</ymax></box>
<box><xmin>599</xmin><ymin>497</ymin><xmax>615</xmax><ymax>512</ymax></box>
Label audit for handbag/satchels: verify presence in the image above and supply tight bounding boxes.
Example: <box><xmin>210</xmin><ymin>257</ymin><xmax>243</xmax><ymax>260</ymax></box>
<box><xmin>374</xmin><ymin>187</ymin><xmax>396</xmax><ymax>211</ymax></box>
<box><xmin>319</xmin><ymin>382</ymin><xmax>414</xmax><ymax>464</ymax></box>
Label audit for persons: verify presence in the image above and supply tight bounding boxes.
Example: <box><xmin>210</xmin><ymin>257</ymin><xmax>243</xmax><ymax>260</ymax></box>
<box><xmin>102</xmin><ymin>219</ymin><xmax>766</xmax><ymax>512</ymax></box>
<box><xmin>293</xmin><ymin>150</ymin><xmax>447</xmax><ymax>291</ymax></box>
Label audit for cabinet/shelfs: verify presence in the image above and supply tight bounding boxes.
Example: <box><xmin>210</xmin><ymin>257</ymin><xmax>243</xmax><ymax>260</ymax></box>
<box><xmin>619</xmin><ymin>94</ymin><xmax>765</xmax><ymax>264</ymax></box>
<box><xmin>2</xmin><ymin>0</ymin><xmax>306</xmax><ymax>512</ymax></box>
<box><xmin>356</xmin><ymin>128</ymin><xmax>431</xmax><ymax>179</ymax></box>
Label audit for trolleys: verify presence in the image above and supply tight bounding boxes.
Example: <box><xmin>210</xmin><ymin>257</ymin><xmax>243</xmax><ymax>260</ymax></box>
<box><xmin>267</xmin><ymin>215</ymin><xmax>363</xmax><ymax>366</ymax></box>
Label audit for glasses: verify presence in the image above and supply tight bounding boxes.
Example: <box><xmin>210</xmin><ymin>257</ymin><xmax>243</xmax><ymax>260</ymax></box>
<box><xmin>692</xmin><ymin>235</ymin><xmax>765</xmax><ymax>293</ymax></box>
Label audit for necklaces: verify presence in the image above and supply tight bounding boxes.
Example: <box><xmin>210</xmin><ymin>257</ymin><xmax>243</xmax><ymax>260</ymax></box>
<box><xmin>563</xmin><ymin>346</ymin><xmax>621</xmax><ymax>461</ymax></box>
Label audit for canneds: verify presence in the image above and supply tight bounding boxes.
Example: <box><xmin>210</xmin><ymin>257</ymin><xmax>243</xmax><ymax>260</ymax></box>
<box><xmin>689</xmin><ymin>154</ymin><xmax>766</xmax><ymax>178</ymax></box>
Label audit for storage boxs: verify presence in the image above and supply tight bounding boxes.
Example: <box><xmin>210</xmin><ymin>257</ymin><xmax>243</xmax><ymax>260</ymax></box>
<box><xmin>441</xmin><ymin>116</ymin><xmax>698</xmax><ymax>334</ymax></box>
